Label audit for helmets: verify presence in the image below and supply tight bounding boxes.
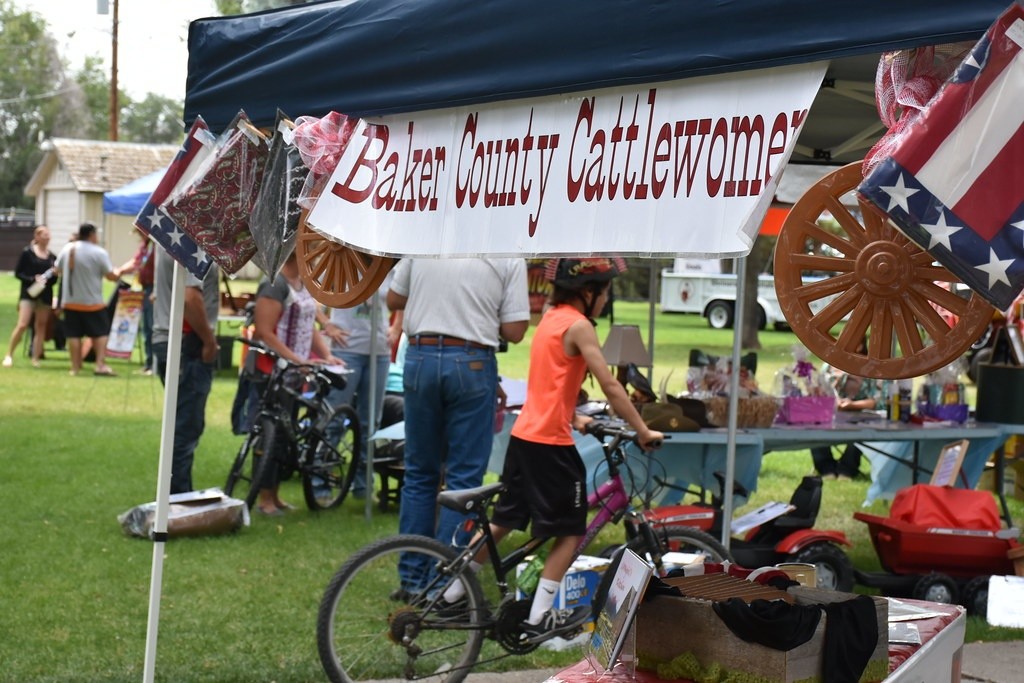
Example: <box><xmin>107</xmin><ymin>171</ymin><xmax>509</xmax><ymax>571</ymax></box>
<box><xmin>545</xmin><ymin>257</ymin><xmax>630</xmax><ymax>279</ymax></box>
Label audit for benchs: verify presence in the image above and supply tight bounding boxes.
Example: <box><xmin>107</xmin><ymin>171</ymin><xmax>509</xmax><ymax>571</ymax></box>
<box><xmin>535</xmin><ymin>592</ymin><xmax>966</xmax><ymax>683</ymax></box>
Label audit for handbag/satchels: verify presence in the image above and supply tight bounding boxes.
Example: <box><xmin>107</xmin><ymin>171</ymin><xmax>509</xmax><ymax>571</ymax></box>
<box><xmin>231</xmin><ymin>376</ymin><xmax>294</xmax><ymax>434</ymax></box>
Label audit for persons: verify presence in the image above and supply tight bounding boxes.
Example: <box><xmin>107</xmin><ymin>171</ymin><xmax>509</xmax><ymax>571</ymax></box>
<box><xmin>808</xmin><ymin>333</ymin><xmax>886</xmax><ymax>484</ymax></box>
<box><xmin>308</xmin><ymin>256</ymin><xmax>406</xmax><ymax>501</ymax></box>
<box><xmin>432</xmin><ymin>257</ymin><xmax>664</xmax><ymax>654</ymax></box>
<box><xmin>377</xmin><ymin>258</ymin><xmax>532</xmax><ymax>609</ymax></box>
<box><xmin>254</xmin><ymin>245</ymin><xmax>347</xmax><ymax>520</ymax></box>
<box><xmin>1</xmin><ymin>223</ymin><xmax>156</xmax><ymax>377</ymax></box>
<box><xmin>151</xmin><ymin>218</ymin><xmax>222</xmax><ymax>493</ymax></box>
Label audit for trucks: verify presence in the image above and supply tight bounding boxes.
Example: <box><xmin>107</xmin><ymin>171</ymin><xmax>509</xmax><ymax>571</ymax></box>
<box><xmin>659</xmin><ymin>258</ymin><xmax>852</xmax><ymax>332</ymax></box>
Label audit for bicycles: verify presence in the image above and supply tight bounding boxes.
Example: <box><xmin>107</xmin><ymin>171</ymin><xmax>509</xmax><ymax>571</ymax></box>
<box><xmin>315</xmin><ymin>418</ymin><xmax>672</xmax><ymax>683</ymax></box>
<box><xmin>224</xmin><ymin>338</ymin><xmax>362</xmax><ymax>530</ymax></box>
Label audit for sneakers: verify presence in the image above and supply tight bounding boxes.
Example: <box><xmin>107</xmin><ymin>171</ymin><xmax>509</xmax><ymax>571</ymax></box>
<box><xmin>432</xmin><ymin>596</ymin><xmax>493</xmax><ymax>622</ymax></box>
<box><xmin>520</xmin><ymin>605</ymin><xmax>592</xmax><ymax>644</ymax></box>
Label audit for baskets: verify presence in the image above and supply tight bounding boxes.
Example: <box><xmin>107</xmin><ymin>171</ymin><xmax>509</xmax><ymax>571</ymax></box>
<box><xmin>680</xmin><ymin>393</ymin><xmax>775</xmax><ymax>428</ymax></box>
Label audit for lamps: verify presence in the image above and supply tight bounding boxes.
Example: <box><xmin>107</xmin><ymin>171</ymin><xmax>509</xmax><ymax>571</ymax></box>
<box><xmin>599</xmin><ymin>323</ymin><xmax>652</xmax><ymax>396</ymax></box>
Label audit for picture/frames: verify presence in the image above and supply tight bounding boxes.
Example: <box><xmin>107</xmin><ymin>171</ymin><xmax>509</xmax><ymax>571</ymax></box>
<box><xmin>928</xmin><ymin>439</ymin><xmax>969</xmax><ymax>489</ymax></box>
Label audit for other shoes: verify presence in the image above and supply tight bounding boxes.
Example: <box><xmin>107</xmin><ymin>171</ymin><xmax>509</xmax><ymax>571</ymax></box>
<box><xmin>256</xmin><ymin>498</ymin><xmax>299</xmax><ymax>517</ymax></box>
<box><xmin>32</xmin><ymin>360</ymin><xmax>39</xmax><ymax>368</ymax></box>
<box><xmin>821</xmin><ymin>466</ymin><xmax>855</xmax><ymax>481</ymax></box>
<box><xmin>390</xmin><ymin>589</ymin><xmax>432</xmax><ymax>609</ymax></box>
<box><xmin>131</xmin><ymin>366</ymin><xmax>153</xmax><ymax>376</ymax></box>
<box><xmin>2</xmin><ymin>356</ymin><xmax>13</xmax><ymax>367</ymax></box>
<box><xmin>94</xmin><ymin>368</ymin><xmax>119</xmax><ymax>377</ymax></box>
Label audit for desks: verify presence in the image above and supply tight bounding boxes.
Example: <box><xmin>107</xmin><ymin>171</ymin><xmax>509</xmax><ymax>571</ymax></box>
<box><xmin>574</xmin><ymin>405</ymin><xmax>1024</xmax><ymax>533</ymax></box>
<box><xmin>217</xmin><ymin>315</ymin><xmax>247</xmax><ymax>376</ymax></box>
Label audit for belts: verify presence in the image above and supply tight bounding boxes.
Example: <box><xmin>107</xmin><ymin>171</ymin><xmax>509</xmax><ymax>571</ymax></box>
<box><xmin>409</xmin><ymin>337</ymin><xmax>488</xmax><ymax>349</ymax></box>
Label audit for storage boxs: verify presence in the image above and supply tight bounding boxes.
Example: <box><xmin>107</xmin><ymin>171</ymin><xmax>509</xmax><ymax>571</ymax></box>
<box><xmin>514</xmin><ymin>555</ymin><xmax>613</xmax><ymax>633</ymax></box>
<box><xmin>781</xmin><ymin>394</ymin><xmax>835</xmax><ymax>423</ymax></box>
<box><xmin>668</xmin><ymin>394</ymin><xmax>779</xmax><ymax>430</ymax></box>
<box><xmin>631</xmin><ymin>573</ymin><xmax>891</xmax><ymax>683</ymax></box>
<box><xmin>919</xmin><ymin>402</ymin><xmax>973</xmax><ymax>422</ymax></box>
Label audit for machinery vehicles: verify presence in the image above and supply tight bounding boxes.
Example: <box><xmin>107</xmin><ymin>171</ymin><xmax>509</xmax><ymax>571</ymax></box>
<box><xmin>625</xmin><ymin>470</ymin><xmax>854</xmax><ymax>594</ymax></box>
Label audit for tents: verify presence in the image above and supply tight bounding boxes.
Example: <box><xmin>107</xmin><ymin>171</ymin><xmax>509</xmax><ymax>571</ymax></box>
<box><xmin>142</xmin><ymin>0</ymin><xmax>1024</xmax><ymax>683</ymax></box>
